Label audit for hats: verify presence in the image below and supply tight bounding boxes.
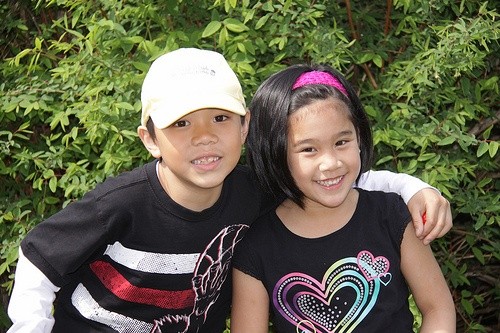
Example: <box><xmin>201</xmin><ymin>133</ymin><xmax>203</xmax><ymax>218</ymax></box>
<box><xmin>139</xmin><ymin>47</ymin><xmax>246</xmax><ymax>130</ymax></box>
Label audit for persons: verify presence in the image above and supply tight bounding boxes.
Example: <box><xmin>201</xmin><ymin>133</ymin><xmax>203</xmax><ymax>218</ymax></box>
<box><xmin>7</xmin><ymin>47</ymin><xmax>453</xmax><ymax>333</ymax></box>
<box><xmin>231</xmin><ymin>65</ymin><xmax>457</xmax><ymax>333</ymax></box>
